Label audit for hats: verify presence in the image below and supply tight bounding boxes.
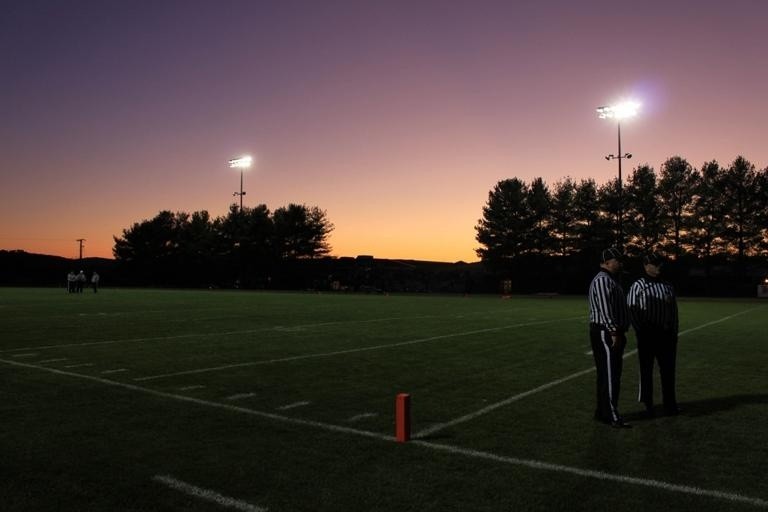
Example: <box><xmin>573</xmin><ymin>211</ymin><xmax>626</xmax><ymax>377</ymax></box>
<box><xmin>643</xmin><ymin>251</ymin><xmax>660</xmax><ymax>265</ymax></box>
<box><xmin>600</xmin><ymin>248</ymin><xmax>623</xmax><ymax>264</ymax></box>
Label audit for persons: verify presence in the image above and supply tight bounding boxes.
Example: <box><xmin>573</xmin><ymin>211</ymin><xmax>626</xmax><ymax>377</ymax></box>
<box><xmin>67</xmin><ymin>270</ymin><xmax>76</xmax><ymax>293</ymax></box>
<box><xmin>588</xmin><ymin>247</ymin><xmax>632</xmax><ymax>428</ymax></box>
<box><xmin>625</xmin><ymin>252</ymin><xmax>684</xmax><ymax>414</ymax></box>
<box><xmin>76</xmin><ymin>270</ymin><xmax>86</xmax><ymax>293</ymax></box>
<box><xmin>91</xmin><ymin>271</ymin><xmax>99</xmax><ymax>293</ymax></box>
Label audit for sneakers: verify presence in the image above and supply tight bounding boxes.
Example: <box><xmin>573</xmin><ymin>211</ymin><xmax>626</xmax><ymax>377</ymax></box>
<box><xmin>640</xmin><ymin>403</ymin><xmax>653</xmax><ymax>412</ymax></box>
<box><xmin>612</xmin><ymin>421</ymin><xmax>632</xmax><ymax>428</ymax></box>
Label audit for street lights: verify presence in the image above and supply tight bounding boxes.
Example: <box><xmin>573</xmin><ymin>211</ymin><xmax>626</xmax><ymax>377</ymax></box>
<box><xmin>229</xmin><ymin>155</ymin><xmax>251</xmax><ymax>214</ymax></box>
<box><xmin>598</xmin><ymin>101</ymin><xmax>638</xmax><ymax>254</ymax></box>
